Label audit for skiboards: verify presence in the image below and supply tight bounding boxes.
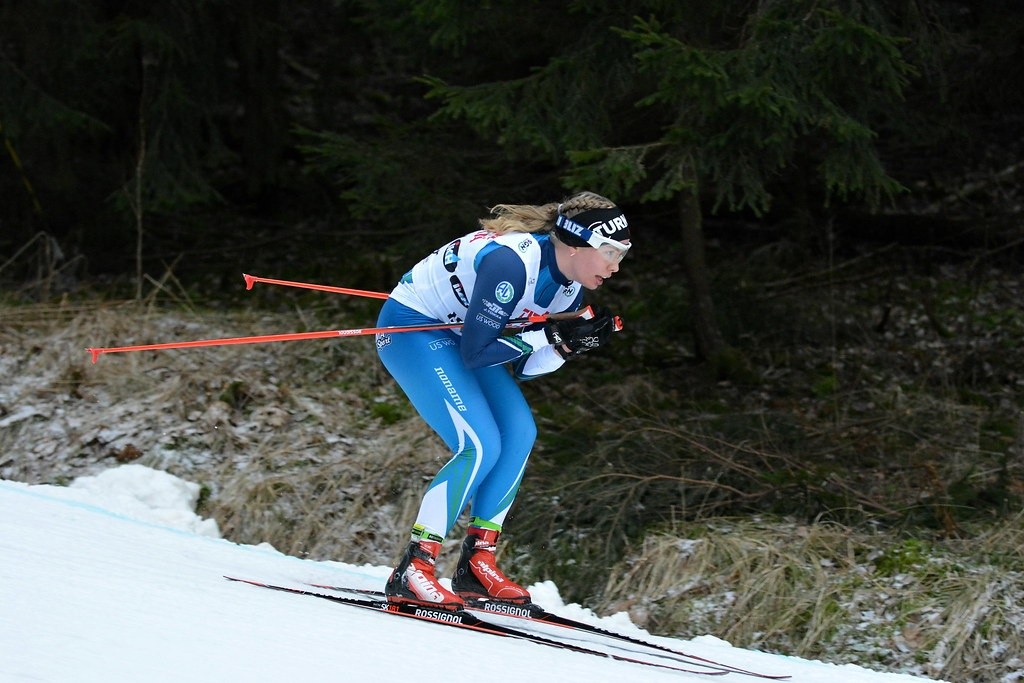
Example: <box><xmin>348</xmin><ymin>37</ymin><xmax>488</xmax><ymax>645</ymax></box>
<box><xmin>219</xmin><ymin>572</ymin><xmax>795</xmax><ymax>683</ymax></box>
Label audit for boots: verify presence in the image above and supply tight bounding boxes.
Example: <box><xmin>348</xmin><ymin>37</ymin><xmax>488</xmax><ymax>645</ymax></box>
<box><xmin>385</xmin><ymin>542</ymin><xmax>465</xmax><ymax>610</ymax></box>
<box><xmin>452</xmin><ymin>526</ymin><xmax>531</xmax><ymax>605</ymax></box>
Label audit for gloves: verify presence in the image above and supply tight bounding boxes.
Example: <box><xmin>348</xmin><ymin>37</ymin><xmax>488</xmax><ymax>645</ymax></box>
<box><xmin>544</xmin><ymin>307</ymin><xmax>612</xmax><ymax>344</ymax></box>
<box><xmin>555</xmin><ymin>319</ymin><xmax>612</xmax><ymax>361</ymax></box>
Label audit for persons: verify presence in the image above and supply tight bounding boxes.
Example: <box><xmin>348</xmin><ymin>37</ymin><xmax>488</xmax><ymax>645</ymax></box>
<box><xmin>373</xmin><ymin>195</ymin><xmax>632</xmax><ymax>613</ymax></box>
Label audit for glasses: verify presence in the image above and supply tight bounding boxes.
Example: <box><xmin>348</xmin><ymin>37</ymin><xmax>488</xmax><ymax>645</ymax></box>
<box><xmin>587</xmin><ymin>231</ymin><xmax>632</xmax><ymax>263</ymax></box>
